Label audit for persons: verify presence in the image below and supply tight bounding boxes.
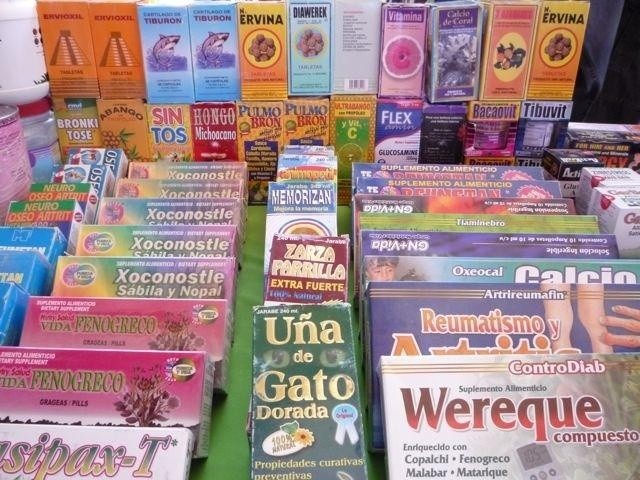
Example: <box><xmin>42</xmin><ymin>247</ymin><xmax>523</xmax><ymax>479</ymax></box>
<box><xmin>365</xmin><ymin>256</ymin><xmax>399</xmax><ymax>282</ymax></box>
<box><xmin>599</xmin><ymin>304</ymin><xmax>640</xmax><ymax>349</ymax></box>
